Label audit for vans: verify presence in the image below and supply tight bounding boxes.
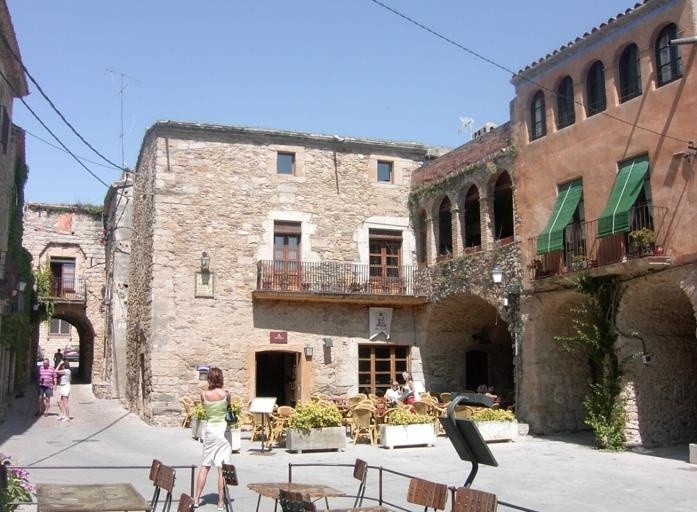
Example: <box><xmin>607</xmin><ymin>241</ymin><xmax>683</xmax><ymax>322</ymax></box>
<box><xmin>63</xmin><ymin>343</ymin><xmax>79</xmax><ymax>361</ymax></box>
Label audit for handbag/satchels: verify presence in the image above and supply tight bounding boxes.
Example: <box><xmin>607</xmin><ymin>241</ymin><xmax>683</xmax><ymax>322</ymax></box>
<box><xmin>59</xmin><ymin>376</ymin><xmax>68</xmax><ymax>385</ymax></box>
<box><xmin>225</xmin><ymin>391</ymin><xmax>238</xmax><ymax>424</ymax></box>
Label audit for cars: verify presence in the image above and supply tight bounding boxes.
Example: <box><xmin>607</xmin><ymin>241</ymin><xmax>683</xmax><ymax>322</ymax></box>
<box><xmin>37</xmin><ymin>346</ymin><xmax>45</xmax><ymax>361</ymax></box>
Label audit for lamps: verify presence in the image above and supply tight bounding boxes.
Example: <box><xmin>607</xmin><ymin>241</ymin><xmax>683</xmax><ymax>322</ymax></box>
<box><xmin>490</xmin><ymin>266</ymin><xmax>523</xmax><ymax>290</ymax></box>
<box><xmin>200</xmin><ymin>251</ymin><xmax>211</xmax><ymax>273</ymax></box>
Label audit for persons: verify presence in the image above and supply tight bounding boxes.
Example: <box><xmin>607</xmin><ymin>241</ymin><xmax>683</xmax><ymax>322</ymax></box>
<box><xmin>54</xmin><ymin>358</ymin><xmax>73</xmax><ymax>421</ymax></box>
<box><xmin>384</xmin><ymin>380</ymin><xmax>402</xmax><ymax>400</ymax></box>
<box><xmin>190</xmin><ymin>367</ymin><xmax>232</xmax><ymax>510</ymax></box>
<box><xmin>401</xmin><ymin>369</ymin><xmax>417</xmax><ymax>415</ymax></box>
<box><xmin>53</xmin><ymin>348</ymin><xmax>62</xmax><ymax>368</ymax></box>
<box><xmin>31</xmin><ymin>358</ymin><xmax>58</xmax><ymax>417</ymax></box>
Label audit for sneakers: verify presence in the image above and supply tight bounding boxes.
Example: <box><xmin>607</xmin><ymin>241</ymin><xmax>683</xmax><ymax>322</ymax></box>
<box><xmin>193</xmin><ymin>498</ymin><xmax>200</xmax><ymax>508</ymax></box>
<box><xmin>217</xmin><ymin>502</ymin><xmax>224</xmax><ymax>511</ymax></box>
<box><xmin>56</xmin><ymin>413</ymin><xmax>73</xmax><ymax>421</ymax></box>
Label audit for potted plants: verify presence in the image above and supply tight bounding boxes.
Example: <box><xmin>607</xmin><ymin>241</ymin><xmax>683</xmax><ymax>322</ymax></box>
<box><xmin>470</xmin><ymin>408</ymin><xmax>518</xmax><ymax>444</ymax></box>
<box><xmin>379</xmin><ymin>405</ymin><xmax>435</xmax><ymax>449</ymax></box>
<box><xmin>286</xmin><ymin>399</ymin><xmax>346</xmax><ymax>454</ymax></box>
<box><xmin>572</xmin><ymin>255</ymin><xmax>598</xmax><ymax>269</ymax></box>
<box><xmin>263</xmin><ymin>272</ymin><xmax>420</xmax><ymax>295</ymax></box>
<box><xmin>627</xmin><ymin>227</ymin><xmax>658</xmax><ymax>257</ymax></box>
<box><xmin>526</xmin><ymin>259</ymin><xmax>545</xmax><ymax>280</ymax></box>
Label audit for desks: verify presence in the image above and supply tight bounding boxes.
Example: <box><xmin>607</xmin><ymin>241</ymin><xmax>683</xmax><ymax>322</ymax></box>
<box><xmin>35</xmin><ymin>483</ymin><xmax>153</xmax><ymax>511</ymax></box>
<box><xmin>245</xmin><ymin>483</ymin><xmax>394</xmax><ymax>512</ymax></box>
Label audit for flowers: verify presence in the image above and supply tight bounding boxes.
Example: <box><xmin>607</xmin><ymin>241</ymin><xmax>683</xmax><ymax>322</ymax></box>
<box><xmin>0</xmin><ymin>453</ymin><xmax>38</xmax><ymax>512</ymax></box>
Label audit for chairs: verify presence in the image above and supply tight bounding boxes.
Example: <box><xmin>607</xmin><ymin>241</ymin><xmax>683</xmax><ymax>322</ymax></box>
<box><xmin>146</xmin><ymin>459</ymin><xmax>194</xmax><ymax>512</ymax></box>
<box><xmin>180</xmin><ymin>396</ymin><xmax>196</xmax><ymax>428</ymax></box>
<box><xmin>230</xmin><ymin>392</ymin><xmax>503</xmax><ymax>447</ymax></box>
<box><xmin>353</xmin><ymin>458</ymin><xmax>368</xmax><ymax>507</ymax></box>
<box><xmin>406</xmin><ymin>478</ymin><xmax>498</xmax><ymax>512</ymax></box>
